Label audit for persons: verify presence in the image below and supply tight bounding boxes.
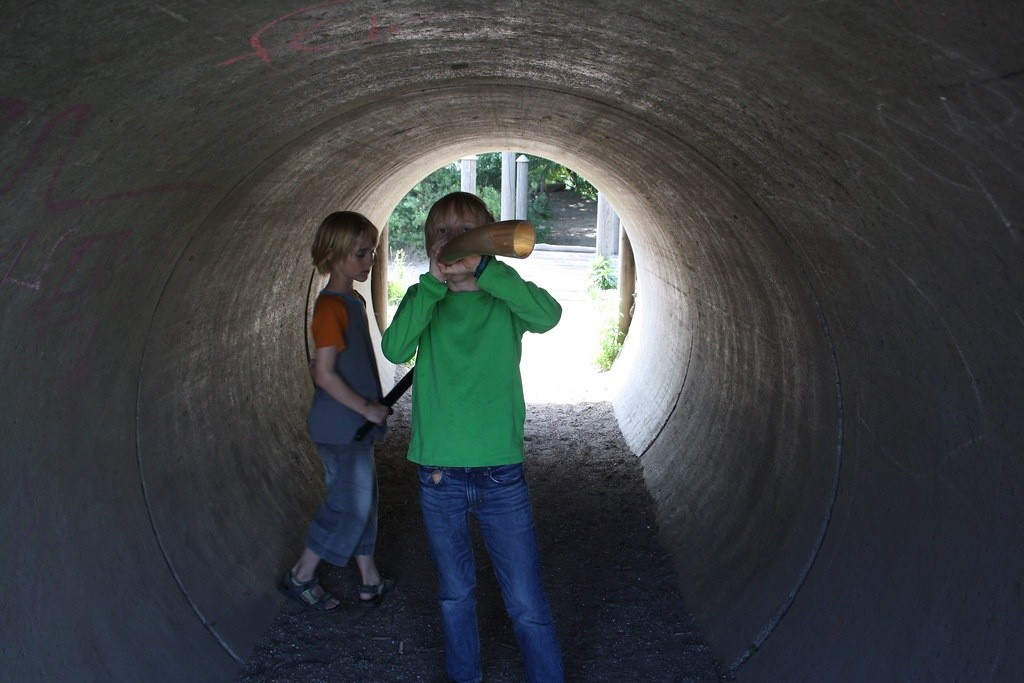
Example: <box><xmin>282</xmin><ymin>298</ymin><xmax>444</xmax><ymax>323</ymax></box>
<box><xmin>382</xmin><ymin>191</ymin><xmax>565</xmax><ymax>683</ymax></box>
<box><xmin>281</xmin><ymin>211</ymin><xmax>397</xmax><ymax>613</ymax></box>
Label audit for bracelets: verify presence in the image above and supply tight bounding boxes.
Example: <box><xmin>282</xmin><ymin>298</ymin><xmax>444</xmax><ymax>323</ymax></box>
<box><xmin>474</xmin><ymin>255</ymin><xmax>491</xmax><ymax>279</ymax></box>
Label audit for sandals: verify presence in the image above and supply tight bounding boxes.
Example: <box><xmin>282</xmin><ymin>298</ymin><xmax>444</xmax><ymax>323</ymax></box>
<box><xmin>352</xmin><ymin>572</ymin><xmax>395</xmax><ymax>607</ymax></box>
<box><xmin>280</xmin><ymin>566</ymin><xmax>342</xmax><ymax>614</ymax></box>
